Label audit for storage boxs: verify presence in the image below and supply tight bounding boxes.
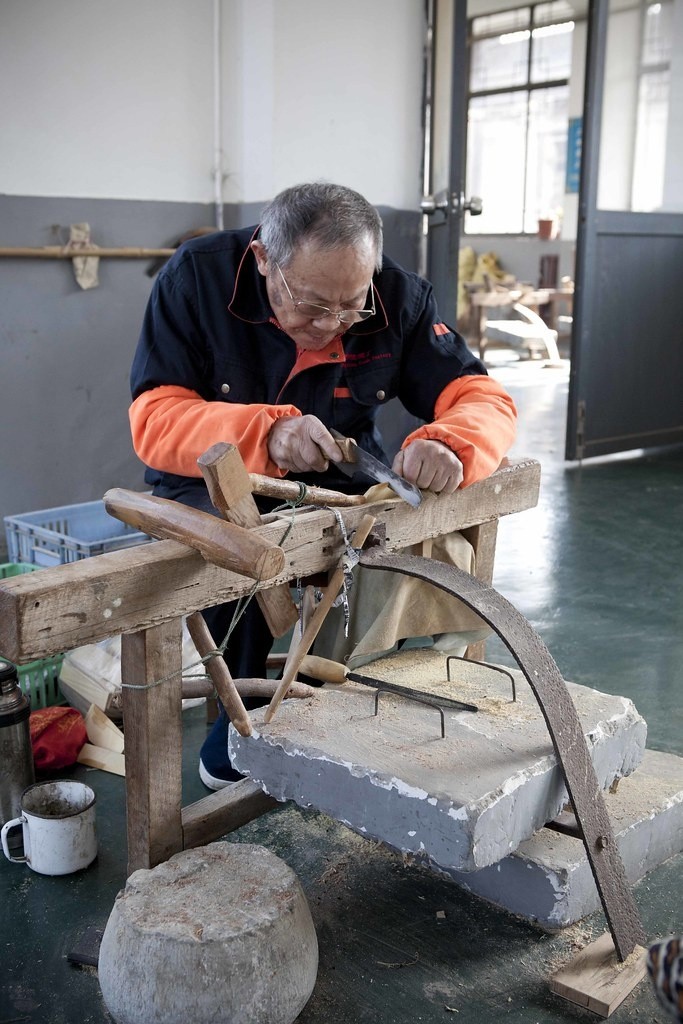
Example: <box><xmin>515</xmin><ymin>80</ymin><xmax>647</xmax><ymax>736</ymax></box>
<box><xmin>1</xmin><ymin>490</ymin><xmax>152</xmax><ymax>710</ymax></box>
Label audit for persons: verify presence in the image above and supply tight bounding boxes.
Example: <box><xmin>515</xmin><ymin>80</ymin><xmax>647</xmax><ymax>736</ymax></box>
<box><xmin>129</xmin><ymin>183</ymin><xmax>517</xmax><ymax>790</ymax></box>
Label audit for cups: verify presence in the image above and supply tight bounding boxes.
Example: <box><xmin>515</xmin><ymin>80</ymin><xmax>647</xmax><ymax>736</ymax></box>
<box><xmin>0</xmin><ymin>779</ymin><xmax>98</xmax><ymax>877</ymax></box>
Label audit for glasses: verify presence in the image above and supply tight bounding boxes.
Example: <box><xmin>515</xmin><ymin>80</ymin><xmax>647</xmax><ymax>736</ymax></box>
<box><xmin>268</xmin><ymin>251</ymin><xmax>376</xmax><ymax>323</ymax></box>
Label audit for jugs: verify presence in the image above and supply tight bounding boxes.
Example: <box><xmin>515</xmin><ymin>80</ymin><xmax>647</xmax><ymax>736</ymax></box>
<box><xmin>0</xmin><ymin>662</ymin><xmax>36</xmax><ymax>849</ymax></box>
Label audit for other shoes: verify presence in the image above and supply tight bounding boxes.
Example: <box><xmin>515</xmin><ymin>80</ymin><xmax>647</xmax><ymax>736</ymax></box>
<box><xmin>198</xmin><ymin>717</ymin><xmax>247</xmax><ymax>791</ymax></box>
